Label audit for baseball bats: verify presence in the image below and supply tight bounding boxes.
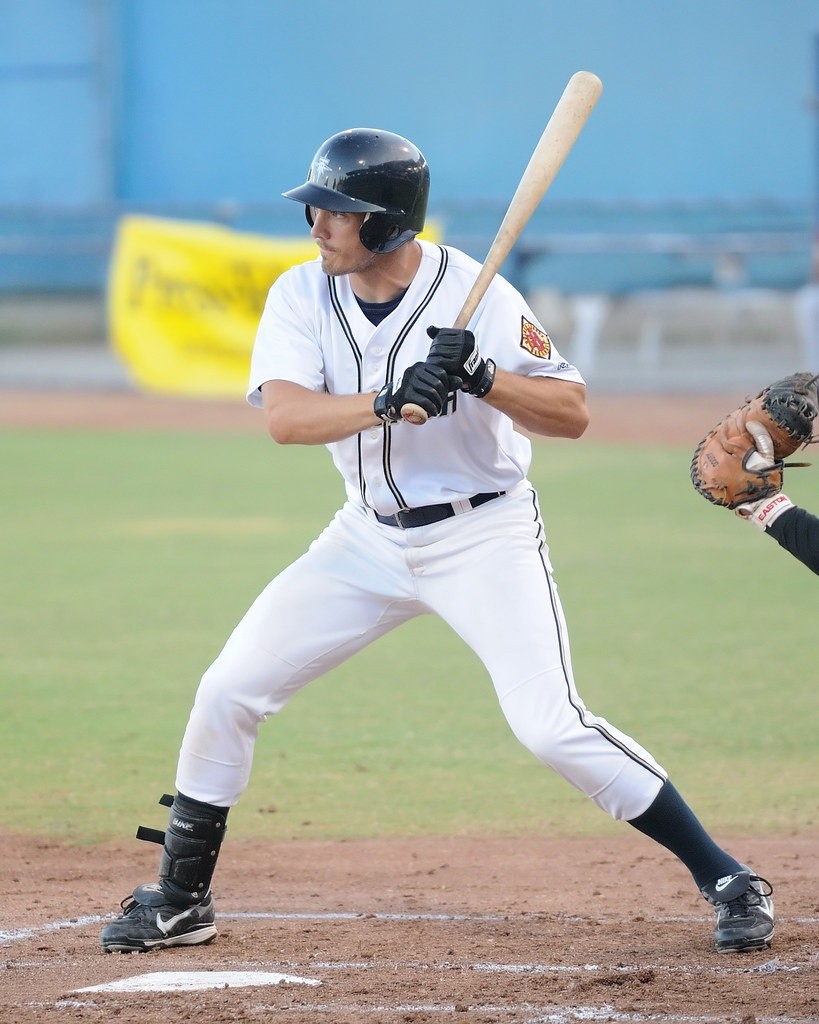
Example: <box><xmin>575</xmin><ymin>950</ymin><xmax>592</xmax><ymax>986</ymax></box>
<box><xmin>400</xmin><ymin>68</ymin><xmax>603</xmax><ymax>427</ymax></box>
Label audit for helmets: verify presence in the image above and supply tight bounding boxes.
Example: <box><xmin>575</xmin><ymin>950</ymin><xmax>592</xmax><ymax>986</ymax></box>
<box><xmin>281</xmin><ymin>128</ymin><xmax>427</xmax><ymax>252</ymax></box>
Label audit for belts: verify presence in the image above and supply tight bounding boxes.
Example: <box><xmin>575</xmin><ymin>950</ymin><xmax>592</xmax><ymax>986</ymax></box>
<box><xmin>375</xmin><ymin>490</ymin><xmax>503</xmax><ymax>530</ymax></box>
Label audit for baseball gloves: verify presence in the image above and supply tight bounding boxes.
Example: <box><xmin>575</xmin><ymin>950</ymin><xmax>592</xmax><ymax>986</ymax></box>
<box><xmin>690</xmin><ymin>371</ymin><xmax>819</xmax><ymax>512</ymax></box>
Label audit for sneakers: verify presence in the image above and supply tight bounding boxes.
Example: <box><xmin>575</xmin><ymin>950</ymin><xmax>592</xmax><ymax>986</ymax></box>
<box><xmin>101</xmin><ymin>879</ymin><xmax>216</xmax><ymax>950</ymax></box>
<box><xmin>701</xmin><ymin>864</ymin><xmax>775</xmax><ymax>953</ymax></box>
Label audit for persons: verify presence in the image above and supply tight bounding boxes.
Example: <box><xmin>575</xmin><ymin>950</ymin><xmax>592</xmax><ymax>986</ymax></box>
<box><xmin>101</xmin><ymin>125</ymin><xmax>777</xmax><ymax>955</ymax></box>
<box><xmin>726</xmin><ymin>419</ymin><xmax>819</xmax><ymax>578</ymax></box>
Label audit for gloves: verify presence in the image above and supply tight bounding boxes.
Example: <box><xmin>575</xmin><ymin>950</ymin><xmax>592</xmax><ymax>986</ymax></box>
<box><xmin>372</xmin><ymin>361</ymin><xmax>463</xmax><ymax>425</ymax></box>
<box><xmin>423</xmin><ymin>326</ymin><xmax>496</xmax><ymax>398</ymax></box>
<box><xmin>727</xmin><ymin>423</ymin><xmax>794</xmax><ymax>532</ymax></box>
